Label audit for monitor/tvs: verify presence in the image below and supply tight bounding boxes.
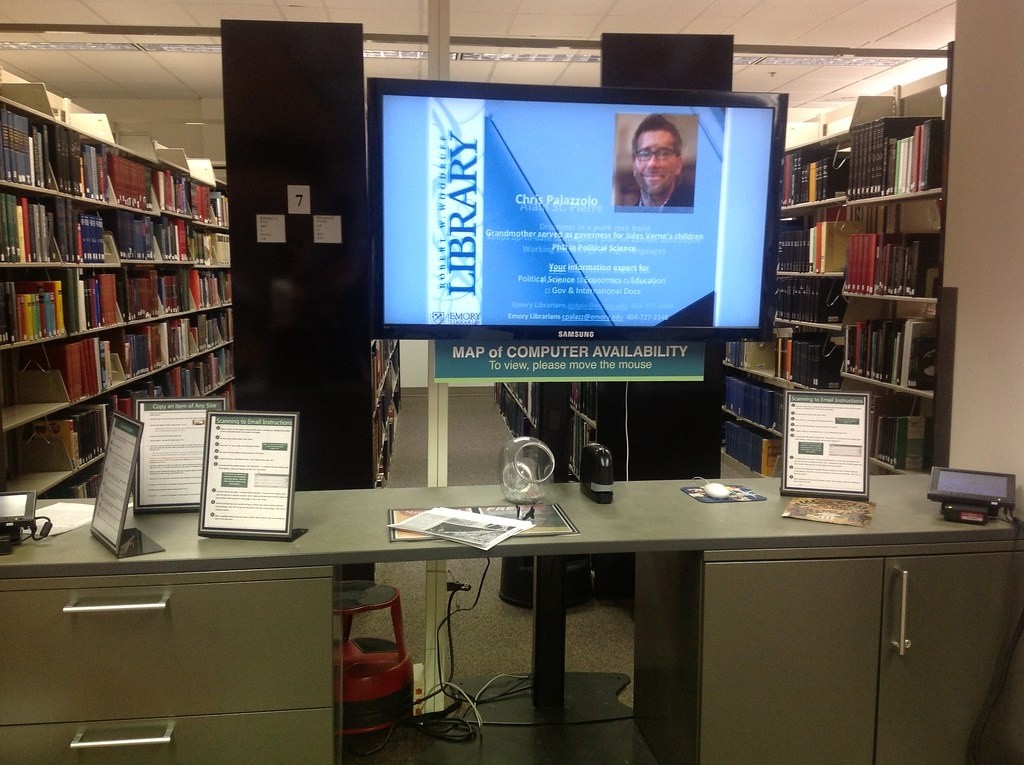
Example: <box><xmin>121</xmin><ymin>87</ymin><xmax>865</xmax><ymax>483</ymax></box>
<box><xmin>367</xmin><ymin>77</ymin><xmax>790</xmax><ymax>338</ymax></box>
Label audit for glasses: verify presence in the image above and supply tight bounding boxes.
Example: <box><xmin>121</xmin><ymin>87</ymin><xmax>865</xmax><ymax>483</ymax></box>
<box><xmin>633</xmin><ymin>147</ymin><xmax>675</xmax><ymax>161</ymax></box>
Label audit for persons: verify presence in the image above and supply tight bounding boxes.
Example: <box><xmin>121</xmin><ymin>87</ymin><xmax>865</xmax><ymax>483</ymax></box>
<box><xmin>632</xmin><ymin>114</ymin><xmax>694</xmax><ymax>207</ymax></box>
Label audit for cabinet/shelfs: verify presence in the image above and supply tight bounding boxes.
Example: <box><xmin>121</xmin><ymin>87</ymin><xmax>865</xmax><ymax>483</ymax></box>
<box><xmin>719</xmin><ymin>113</ymin><xmax>952</xmax><ymax>479</ymax></box>
<box><xmin>220</xmin><ymin>18</ymin><xmax>401</xmax><ymax>590</ymax></box>
<box><xmin>0</xmin><ymin>470</ymin><xmax>1024</xmax><ymax>765</ymax></box>
<box><xmin>0</xmin><ymin>93</ymin><xmax>235</xmax><ymax>497</ymax></box>
<box><xmin>495</xmin><ymin>34</ymin><xmax>737</xmax><ymax>605</ymax></box>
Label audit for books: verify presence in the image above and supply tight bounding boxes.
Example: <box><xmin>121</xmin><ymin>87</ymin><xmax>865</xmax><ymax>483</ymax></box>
<box><xmin>0</xmin><ymin>105</ymin><xmax>234</xmax><ymax>349</ymax></box>
<box><xmin>493</xmin><ymin>383</ymin><xmax>539</xmax><ymax>437</ymax></box>
<box><xmin>20</xmin><ymin>306</ymin><xmax>234</xmax><ymax>498</ymax></box>
<box><xmin>724</xmin><ymin>339</ymin><xmax>776</xmax><ymax>370</ymax></box>
<box><xmin>724</xmin><ymin>421</ymin><xmax>784</xmax><ymax>476</ymax></box>
<box><xmin>372</xmin><ymin>339</ymin><xmax>402</xmax><ymax>487</ymax></box>
<box><xmin>567</xmin><ymin>381</ymin><xmax>597</xmax><ymax>480</ymax></box>
<box><xmin>781</xmin><ymin>114</ymin><xmax>942</xmax><ymax>207</ymax></box>
<box><xmin>872</xmin><ymin>396</ymin><xmax>933</xmax><ymax>470</ymax></box>
<box><xmin>725</xmin><ymin>373</ymin><xmax>783</xmax><ymax>428</ymax></box>
<box><xmin>777</xmin><ymin>222</ymin><xmax>938</xmax><ymax>389</ymax></box>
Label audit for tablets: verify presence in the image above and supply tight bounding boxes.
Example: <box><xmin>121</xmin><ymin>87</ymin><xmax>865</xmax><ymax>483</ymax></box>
<box><xmin>927</xmin><ymin>467</ymin><xmax>1016</xmax><ymax>508</ymax></box>
<box><xmin>0</xmin><ymin>490</ymin><xmax>36</xmax><ymax>523</ymax></box>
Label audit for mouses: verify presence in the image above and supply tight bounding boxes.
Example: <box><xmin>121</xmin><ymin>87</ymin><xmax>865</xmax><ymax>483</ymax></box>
<box><xmin>705</xmin><ymin>483</ymin><xmax>729</xmax><ymax>499</ymax></box>
